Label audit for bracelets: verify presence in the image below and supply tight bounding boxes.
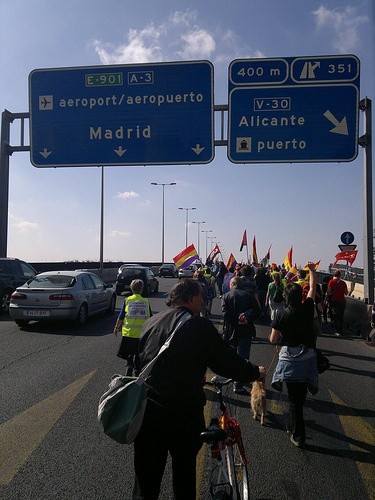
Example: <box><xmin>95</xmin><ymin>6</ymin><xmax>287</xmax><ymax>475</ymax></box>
<box><xmin>116</xmin><ymin>326</ymin><xmax>119</xmax><ymax>329</ymax></box>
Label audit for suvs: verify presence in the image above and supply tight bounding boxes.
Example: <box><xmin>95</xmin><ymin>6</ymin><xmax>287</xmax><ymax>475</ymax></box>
<box><xmin>0</xmin><ymin>256</ymin><xmax>42</xmax><ymax>322</ymax></box>
<box><xmin>115</xmin><ymin>264</ymin><xmax>160</xmax><ymax>298</ymax></box>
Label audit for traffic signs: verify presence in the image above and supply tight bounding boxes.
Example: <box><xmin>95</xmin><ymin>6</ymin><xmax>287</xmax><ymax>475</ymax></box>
<box><xmin>230</xmin><ymin>55</ymin><xmax>358</xmax><ymax>163</ymax></box>
<box><xmin>28</xmin><ymin>60</ymin><xmax>215</xmax><ymax>166</ymax></box>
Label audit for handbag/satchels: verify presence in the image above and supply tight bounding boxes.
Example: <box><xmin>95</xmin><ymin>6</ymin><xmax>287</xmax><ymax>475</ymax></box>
<box><xmin>316</xmin><ymin>351</ymin><xmax>330</xmax><ymax>374</ymax></box>
<box><xmin>97</xmin><ymin>374</ymin><xmax>147</xmax><ymax>445</ymax></box>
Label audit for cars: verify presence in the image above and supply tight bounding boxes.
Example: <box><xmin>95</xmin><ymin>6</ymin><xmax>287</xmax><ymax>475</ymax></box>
<box><xmin>178</xmin><ymin>265</ymin><xmax>198</xmax><ymax>279</ymax></box>
<box><xmin>158</xmin><ymin>264</ymin><xmax>178</xmax><ymax>277</ymax></box>
<box><xmin>10</xmin><ymin>270</ymin><xmax>117</xmax><ymax>329</ymax></box>
<box><xmin>193</xmin><ymin>263</ymin><xmax>205</xmax><ymax>270</ymax></box>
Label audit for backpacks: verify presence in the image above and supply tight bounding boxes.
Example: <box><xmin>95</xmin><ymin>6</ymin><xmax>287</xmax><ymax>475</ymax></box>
<box><xmin>273</xmin><ymin>288</ymin><xmax>284</xmax><ymax>303</ymax></box>
<box><xmin>206</xmin><ymin>276</ymin><xmax>213</xmax><ymax>285</ymax></box>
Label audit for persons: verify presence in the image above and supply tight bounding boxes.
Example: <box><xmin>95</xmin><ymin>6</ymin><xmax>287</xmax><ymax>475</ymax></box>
<box><xmin>114</xmin><ymin>262</ymin><xmax>349</xmax><ymax>500</ymax></box>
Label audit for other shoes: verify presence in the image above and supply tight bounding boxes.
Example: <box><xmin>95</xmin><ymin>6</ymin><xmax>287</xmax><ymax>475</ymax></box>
<box><xmin>232</xmin><ymin>383</ymin><xmax>245</xmax><ymax>392</ymax></box>
<box><xmin>219</xmin><ymin>295</ymin><xmax>222</xmax><ymax>299</ymax></box>
<box><xmin>286</xmin><ymin>425</ymin><xmax>302</xmax><ymax>447</ymax></box>
<box><xmin>125</xmin><ymin>368</ymin><xmax>140</xmax><ymax>377</ymax></box>
<box><xmin>335</xmin><ymin>331</ymin><xmax>344</xmax><ymax>336</ymax></box>
<box><xmin>318</xmin><ymin>322</ymin><xmax>323</xmax><ymax>329</ymax></box>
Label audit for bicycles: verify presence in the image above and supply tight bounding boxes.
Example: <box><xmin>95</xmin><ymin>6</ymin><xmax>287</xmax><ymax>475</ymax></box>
<box><xmin>199</xmin><ymin>372</ymin><xmax>254</xmax><ymax>500</ymax></box>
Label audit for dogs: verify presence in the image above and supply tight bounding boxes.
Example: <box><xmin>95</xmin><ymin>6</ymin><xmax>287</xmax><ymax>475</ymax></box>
<box><xmin>251</xmin><ymin>372</ymin><xmax>267</xmax><ymax>425</ymax></box>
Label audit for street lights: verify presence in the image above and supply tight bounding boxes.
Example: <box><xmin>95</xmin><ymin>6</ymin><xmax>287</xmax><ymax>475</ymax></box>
<box><xmin>192</xmin><ymin>221</ymin><xmax>228</xmax><ymax>265</ymax></box>
<box><xmin>151</xmin><ymin>181</ymin><xmax>178</xmax><ymax>264</ymax></box>
<box><xmin>178</xmin><ymin>207</ymin><xmax>197</xmax><ymax>248</ymax></box>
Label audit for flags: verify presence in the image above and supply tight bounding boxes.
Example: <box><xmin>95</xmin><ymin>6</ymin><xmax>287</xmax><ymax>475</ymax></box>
<box><xmin>173</xmin><ymin>229</ymin><xmax>358</xmax><ymax>281</ymax></box>
